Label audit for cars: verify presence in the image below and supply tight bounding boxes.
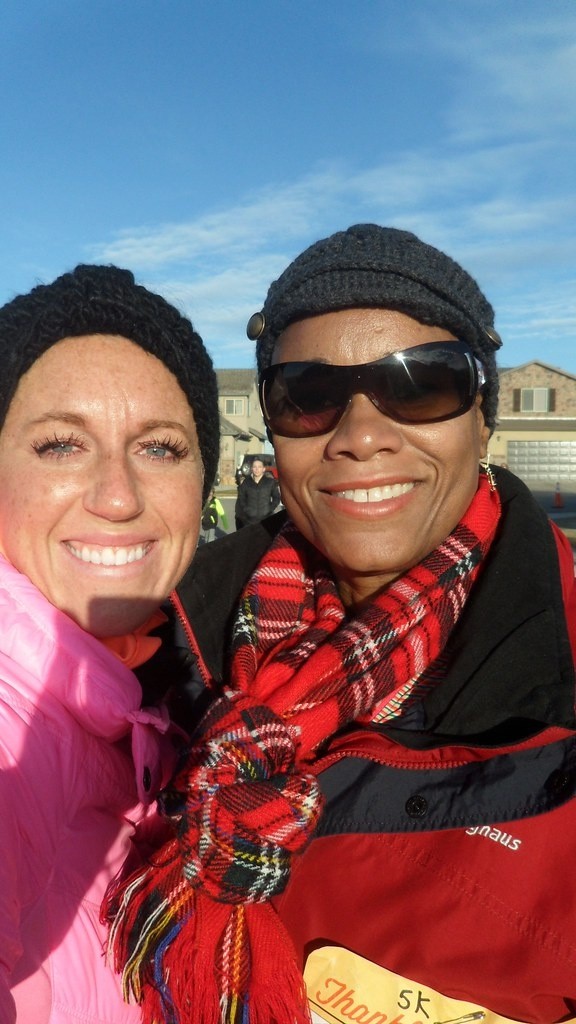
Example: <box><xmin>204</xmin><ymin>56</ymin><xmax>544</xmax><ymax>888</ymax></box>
<box><xmin>235</xmin><ymin>452</ymin><xmax>279</xmax><ymax>486</ymax></box>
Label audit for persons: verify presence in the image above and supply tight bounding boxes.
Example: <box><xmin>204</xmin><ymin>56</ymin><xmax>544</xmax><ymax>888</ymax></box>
<box><xmin>2</xmin><ymin>264</ymin><xmax>248</xmax><ymax>1023</ymax></box>
<box><xmin>201</xmin><ymin>487</ymin><xmax>230</xmax><ymax>544</ymax></box>
<box><xmin>101</xmin><ymin>223</ymin><xmax>576</xmax><ymax>1024</ymax></box>
<box><xmin>234</xmin><ymin>459</ymin><xmax>282</xmax><ymax>533</ymax></box>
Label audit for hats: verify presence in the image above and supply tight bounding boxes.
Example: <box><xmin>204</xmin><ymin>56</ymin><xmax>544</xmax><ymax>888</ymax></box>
<box><xmin>249</xmin><ymin>224</ymin><xmax>502</xmax><ymax>428</ymax></box>
<box><xmin>4</xmin><ymin>266</ymin><xmax>219</xmax><ymax>509</ymax></box>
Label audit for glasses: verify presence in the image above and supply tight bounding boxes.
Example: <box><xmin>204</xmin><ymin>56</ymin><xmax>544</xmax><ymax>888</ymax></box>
<box><xmin>259</xmin><ymin>341</ymin><xmax>487</xmax><ymax>437</ymax></box>
